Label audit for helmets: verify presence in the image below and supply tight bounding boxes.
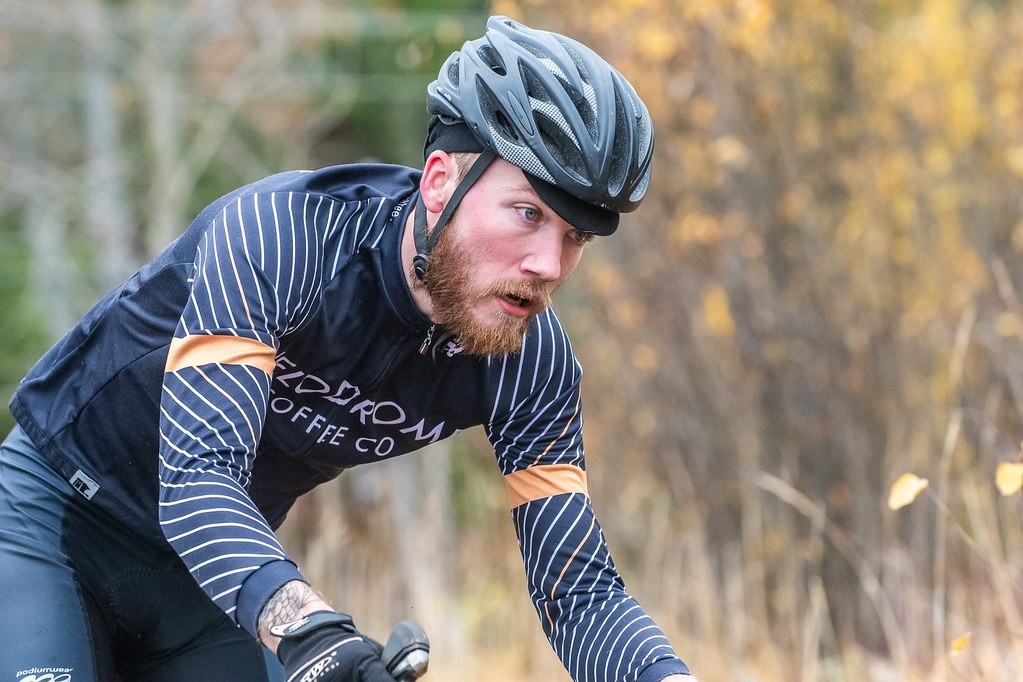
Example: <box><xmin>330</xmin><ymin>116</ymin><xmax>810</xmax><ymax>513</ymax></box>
<box><xmin>425</xmin><ymin>14</ymin><xmax>656</xmax><ymax>213</ymax></box>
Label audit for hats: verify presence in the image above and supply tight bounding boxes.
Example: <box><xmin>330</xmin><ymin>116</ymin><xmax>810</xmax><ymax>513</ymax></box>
<box><xmin>422</xmin><ymin>114</ymin><xmax>621</xmax><ymax>236</ymax></box>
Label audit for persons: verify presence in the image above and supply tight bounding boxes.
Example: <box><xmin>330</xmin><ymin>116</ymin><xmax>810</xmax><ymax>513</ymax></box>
<box><xmin>0</xmin><ymin>14</ymin><xmax>699</xmax><ymax>682</ymax></box>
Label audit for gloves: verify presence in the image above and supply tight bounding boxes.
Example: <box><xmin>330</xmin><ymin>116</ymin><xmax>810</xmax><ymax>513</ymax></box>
<box><xmin>267</xmin><ymin>610</ymin><xmax>412</xmax><ymax>682</ymax></box>
